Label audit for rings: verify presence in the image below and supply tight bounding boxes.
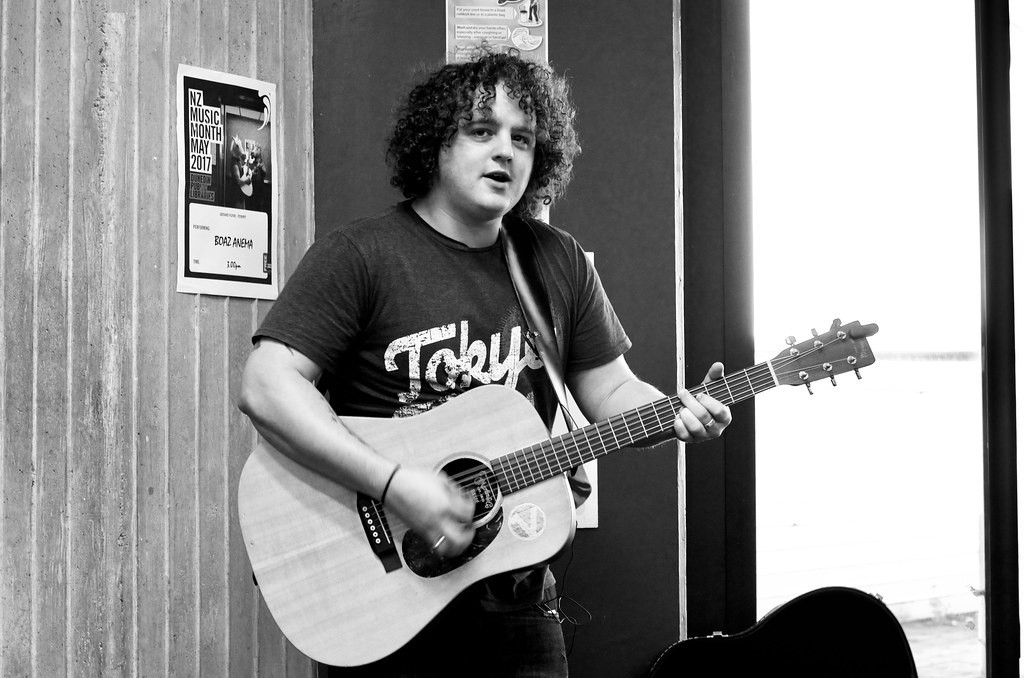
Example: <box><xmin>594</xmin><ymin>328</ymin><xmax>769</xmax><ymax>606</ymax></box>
<box><xmin>705</xmin><ymin>419</ymin><xmax>716</xmax><ymax>429</ymax></box>
<box><xmin>434</xmin><ymin>536</ymin><xmax>445</xmax><ymax>548</ymax></box>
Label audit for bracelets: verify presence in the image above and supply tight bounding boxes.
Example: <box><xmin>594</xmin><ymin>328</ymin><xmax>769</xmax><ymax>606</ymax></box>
<box><xmin>381</xmin><ymin>464</ymin><xmax>401</xmax><ymax>504</ymax></box>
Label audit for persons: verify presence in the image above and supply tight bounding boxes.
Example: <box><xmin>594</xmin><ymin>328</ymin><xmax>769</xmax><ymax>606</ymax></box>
<box><xmin>241</xmin><ymin>52</ymin><xmax>732</xmax><ymax>678</ymax></box>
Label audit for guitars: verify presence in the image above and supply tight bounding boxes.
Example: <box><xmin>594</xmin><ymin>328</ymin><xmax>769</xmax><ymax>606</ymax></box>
<box><xmin>236</xmin><ymin>322</ymin><xmax>877</xmax><ymax>667</ymax></box>
